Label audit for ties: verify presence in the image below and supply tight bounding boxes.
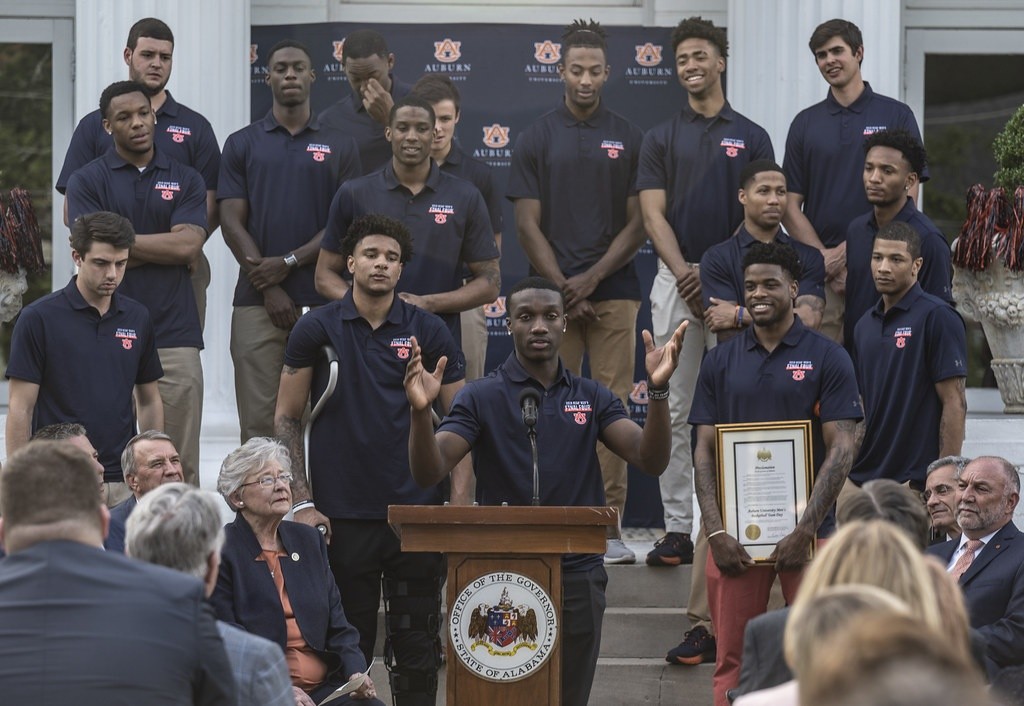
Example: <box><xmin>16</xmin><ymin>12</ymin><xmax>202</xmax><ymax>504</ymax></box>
<box><xmin>950</xmin><ymin>539</ymin><xmax>985</xmax><ymax>583</ymax></box>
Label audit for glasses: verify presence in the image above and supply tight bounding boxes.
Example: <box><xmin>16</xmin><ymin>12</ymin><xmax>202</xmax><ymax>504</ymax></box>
<box><xmin>920</xmin><ymin>485</ymin><xmax>956</xmax><ymax>504</ymax></box>
<box><xmin>241</xmin><ymin>472</ymin><xmax>292</xmax><ymax>489</ymax></box>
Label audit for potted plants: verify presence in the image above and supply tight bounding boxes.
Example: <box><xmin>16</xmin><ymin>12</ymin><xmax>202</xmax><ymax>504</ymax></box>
<box><xmin>951</xmin><ymin>103</ymin><xmax>1024</xmax><ymax>412</ymax></box>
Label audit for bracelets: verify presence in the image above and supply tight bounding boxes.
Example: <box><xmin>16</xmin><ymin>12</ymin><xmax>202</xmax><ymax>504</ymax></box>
<box><xmin>734</xmin><ymin>303</ymin><xmax>738</xmax><ymax>326</ymax></box>
<box><xmin>292</xmin><ymin>499</ymin><xmax>314</xmax><ymax>509</ymax></box>
<box><xmin>706</xmin><ymin>529</ymin><xmax>726</xmax><ymax>540</ymax></box>
<box><xmin>737</xmin><ymin>304</ymin><xmax>743</xmax><ymax>327</ymax></box>
<box><xmin>293</xmin><ymin>502</ymin><xmax>315</xmax><ymax>514</ymax></box>
<box><xmin>647</xmin><ymin>380</ymin><xmax>670</xmax><ymax>401</ymax></box>
<box><xmin>282</xmin><ymin>250</ymin><xmax>300</xmax><ymax>273</ymax></box>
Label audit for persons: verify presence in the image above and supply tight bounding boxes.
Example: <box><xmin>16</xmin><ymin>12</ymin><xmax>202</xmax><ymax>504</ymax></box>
<box><xmin>6</xmin><ymin>210</ymin><xmax>166</xmax><ymax>507</ymax></box>
<box><xmin>665</xmin><ymin>124</ymin><xmax>969</xmax><ymax>669</ymax></box>
<box><xmin>314</xmin><ymin>29</ymin><xmax>502</xmax><ymax>507</ymax></box>
<box><xmin>0</xmin><ymin>424</ymin><xmax>387</xmax><ymax>706</ymax></box>
<box><xmin>515</xmin><ymin>19</ymin><xmax>648</xmax><ymax>563</ymax></box>
<box><xmin>692</xmin><ymin>244</ymin><xmax>858</xmax><ymax>706</ymax></box>
<box><xmin>65</xmin><ymin>82</ymin><xmax>209</xmax><ymax>490</ymax></box>
<box><xmin>272</xmin><ymin>214</ymin><xmax>476</xmax><ymax>705</ymax></box>
<box><xmin>723</xmin><ymin>456</ymin><xmax>1024</xmax><ymax>706</ymax></box>
<box><xmin>780</xmin><ymin>19</ymin><xmax>924</xmax><ymax>347</ymax></box>
<box><xmin>404</xmin><ymin>277</ymin><xmax>690</xmax><ymax>706</ymax></box>
<box><xmin>215</xmin><ymin>38</ymin><xmax>362</xmax><ymax>453</ymax></box>
<box><xmin>636</xmin><ymin>15</ymin><xmax>775</xmax><ymax>565</ymax></box>
<box><xmin>55</xmin><ymin>18</ymin><xmax>223</xmax><ymax>332</ymax></box>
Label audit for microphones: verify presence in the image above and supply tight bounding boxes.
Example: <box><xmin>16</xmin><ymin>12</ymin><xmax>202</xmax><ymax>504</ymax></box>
<box><xmin>518</xmin><ymin>387</ymin><xmax>542</xmax><ymax>427</ymax></box>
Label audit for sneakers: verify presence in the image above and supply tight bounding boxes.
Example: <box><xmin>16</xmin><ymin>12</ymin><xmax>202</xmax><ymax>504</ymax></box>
<box><xmin>645</xmin><ymin>532</ymin><xmax>694</xmax><ymax>566</ymax></box>
<box><xmin>605</xmin><ymin>536</ymin><xmax>636</xmax><ymax>563</ymax></box>
<box><xmin>665</xmin><ymin>625</ymin><xmax>716</xmax><ymax>663</ymax></box>
